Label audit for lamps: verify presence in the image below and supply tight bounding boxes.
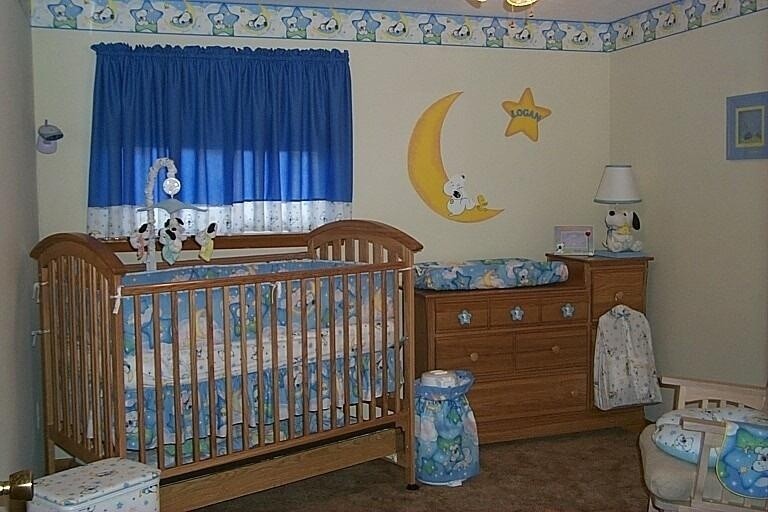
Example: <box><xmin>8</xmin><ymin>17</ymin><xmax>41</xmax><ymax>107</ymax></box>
<box><xmin>592</xmin><ymin>166</ymin><xmax>643</xmax><ymax>251</ymax></box>
<box><xmin>473</xmin><ymin>1</ymin><xmax>539</xmax><ymax>29</ymax></box>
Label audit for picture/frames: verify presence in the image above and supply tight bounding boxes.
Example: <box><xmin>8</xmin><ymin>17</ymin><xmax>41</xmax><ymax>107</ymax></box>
<box><xmin>725</xmin><ymin>91</ymin><xmax>767</xmax><ymax>160</ymax></box>
<box><xmin>553</xmin><ymin>226</ymin><xmax>595</xmax><ymax>258</ymax></box>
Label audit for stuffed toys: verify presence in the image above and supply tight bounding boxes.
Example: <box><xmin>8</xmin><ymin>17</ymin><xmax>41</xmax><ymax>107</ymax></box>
<box><xmin>128</xmin><ymin>156</ymin><xmax>218</xmax><ymax>272</ymax></box>
<box><xmin>601</xmin><ymin>208</ymin><xmax>644</xmax><ymax>255</ymax></box>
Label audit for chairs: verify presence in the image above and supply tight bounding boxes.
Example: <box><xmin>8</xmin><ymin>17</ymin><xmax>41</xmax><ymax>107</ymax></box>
<box><xmin>639</xmin><ymin>376</ymin><xmax>768</xmax><ymax>511</ymax></box>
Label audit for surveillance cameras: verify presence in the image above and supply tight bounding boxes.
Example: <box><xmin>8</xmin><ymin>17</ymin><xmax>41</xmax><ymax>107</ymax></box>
<box><xmin>39</xmin><ymin>124</ymin><xmax>65</xmax><ymax>142</ymax></box>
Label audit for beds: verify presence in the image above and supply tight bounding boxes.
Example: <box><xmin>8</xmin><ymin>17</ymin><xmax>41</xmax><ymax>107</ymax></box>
<box><xmin>29</xmin><ymin>219</ymin><xmax>424</xmax><ymax>512</ymax></box>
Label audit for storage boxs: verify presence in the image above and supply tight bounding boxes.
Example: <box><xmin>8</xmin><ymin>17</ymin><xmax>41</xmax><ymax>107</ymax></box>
<box><xmin>27</xmin><ymin>457</ymin><xmax>162</xmax><ymax>512</ymax></box>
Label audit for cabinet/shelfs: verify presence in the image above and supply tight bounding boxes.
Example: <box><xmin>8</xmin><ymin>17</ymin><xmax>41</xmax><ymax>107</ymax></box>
<box><xmin>397</xmin><ymin>253</ymin><xmax>654</xmax><ymax>445</ymax></box>
